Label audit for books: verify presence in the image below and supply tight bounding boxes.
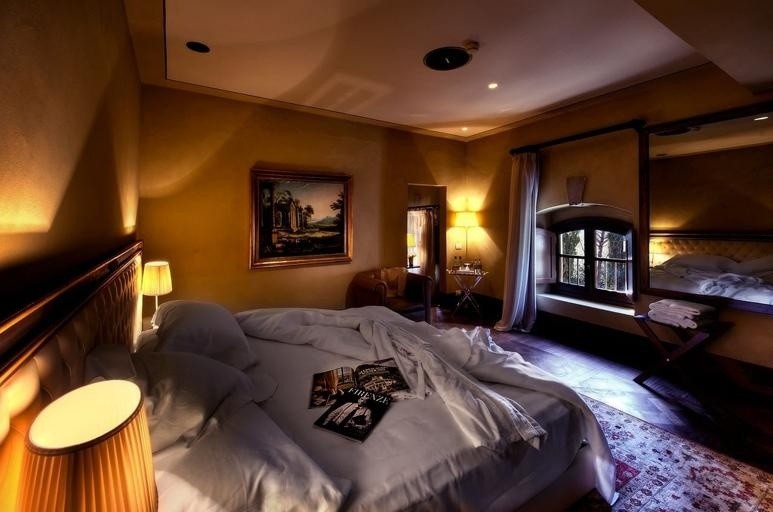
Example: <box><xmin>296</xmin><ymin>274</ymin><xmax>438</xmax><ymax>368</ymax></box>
<box><xmin>313</xmin><ymin>386</ymin><xmax>393</xmax><ymax>445</ymax></box>
<box><xmin>308</xmin><ymin>357</ymin><xmax>410</xmax><ymax>410</ymax></box>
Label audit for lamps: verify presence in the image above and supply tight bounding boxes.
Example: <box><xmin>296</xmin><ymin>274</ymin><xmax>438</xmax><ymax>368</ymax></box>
<box><xmin>14</xmin><ymin>378</ymin><xmax>157</xmax><ymax>512</ymax></box>
<box><xmin>455</xmin><ymin>211</ymin><xmax>480</xmax><ymax>267</ymax></box>
<box><xmin>141</xmin><ymin>261</ymin><xmax>173</xmax><ymax>329</ymax></box>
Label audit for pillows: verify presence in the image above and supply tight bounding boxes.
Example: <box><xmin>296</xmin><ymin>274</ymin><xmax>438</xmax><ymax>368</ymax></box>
<box><xmin>151</xmin><ymin>300</ymin><xmax>258</xmax><ymax>369</ymax></box>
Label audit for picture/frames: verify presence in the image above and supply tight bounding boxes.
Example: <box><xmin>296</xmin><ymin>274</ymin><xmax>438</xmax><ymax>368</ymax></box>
<box><xmin>245</xmin><ymin>167</ymin><xmax>355</xmax><ymax>272</ymax></box>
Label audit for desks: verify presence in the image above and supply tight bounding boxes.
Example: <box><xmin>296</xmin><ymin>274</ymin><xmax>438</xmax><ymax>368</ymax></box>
<box><xmin>633</xmin><ymin>313</ymin><xmax>735</xmax><ymax>384</ymax></box>
<box><xmin>446</xmin><ymin>268</ymin><xmax>488</xmax><ymax>316</ymax></box>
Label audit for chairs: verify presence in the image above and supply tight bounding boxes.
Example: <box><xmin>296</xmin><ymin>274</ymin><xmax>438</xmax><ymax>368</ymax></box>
<box><xmin>346</xmin><ymin>267</ymin><xmax>434</xmax><ymax>325</ymax></box>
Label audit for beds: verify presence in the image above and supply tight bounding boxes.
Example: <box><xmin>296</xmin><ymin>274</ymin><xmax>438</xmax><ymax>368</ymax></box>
<box><xmin>0</xmin><ymin>239</ymin><xmax>620</xmax><ymax>512</ymax></box>
<box><xmin>649</xmin><ymin>238</ymin><xmax>773</xmax><ymax>307</ymax></box>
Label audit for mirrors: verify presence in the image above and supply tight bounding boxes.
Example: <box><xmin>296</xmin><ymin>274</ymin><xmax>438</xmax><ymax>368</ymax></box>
<box><xmin>649</xmin><ymin>230</ymin><xmax>772</xmax><ymax>315</ymax></box>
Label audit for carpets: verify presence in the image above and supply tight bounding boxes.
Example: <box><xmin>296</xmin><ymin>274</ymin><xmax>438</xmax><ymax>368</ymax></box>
<box><xmin>571</xmin><ymin>387</ymin><xmax>773</xmax><ymax>512</ymax></box>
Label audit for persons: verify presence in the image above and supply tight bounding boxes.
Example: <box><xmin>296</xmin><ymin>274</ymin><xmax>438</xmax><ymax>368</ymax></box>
<box><xmin>323</xmin><ymin>395</ymin><xmax>372</xmax><ymax>434</ymax></box>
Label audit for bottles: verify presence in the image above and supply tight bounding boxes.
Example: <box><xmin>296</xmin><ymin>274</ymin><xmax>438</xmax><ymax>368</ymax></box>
<box><xmin>452</xmin><ymin>255</ymin><xmax>463</xmax><ymax>274</ymax></box>
<box><xmin>473</xmin><ymin>258</ymin><xmax>482</xmax><ymax>275</ymax></box>
